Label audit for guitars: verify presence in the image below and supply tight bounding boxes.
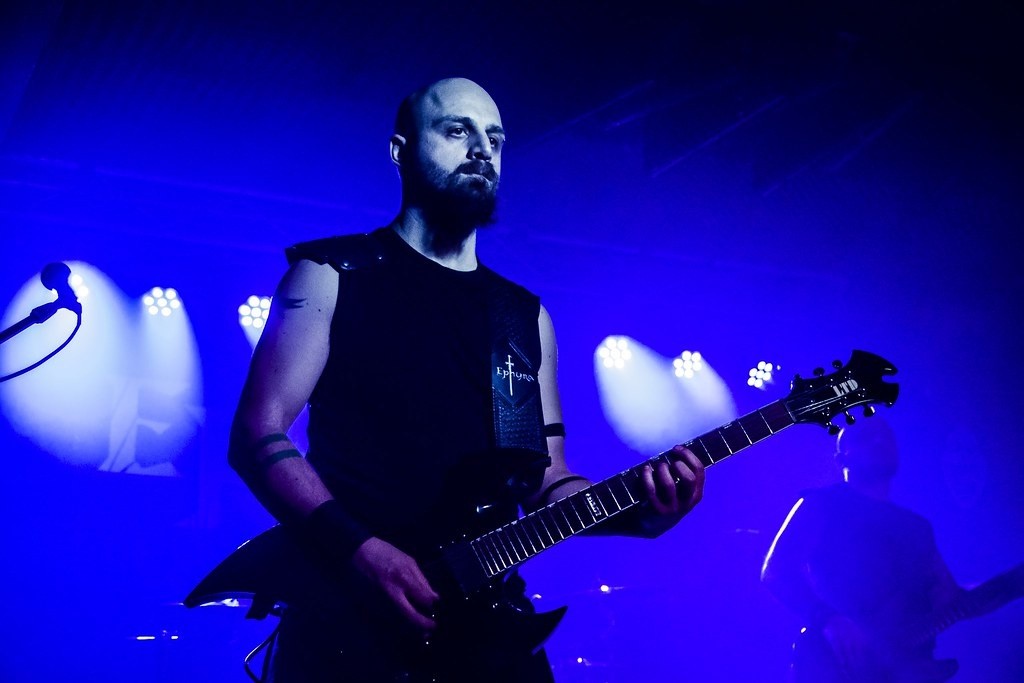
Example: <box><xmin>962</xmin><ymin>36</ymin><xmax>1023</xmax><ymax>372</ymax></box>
<box><xmin>178</xmin><ymin>343</ymin><xmax>906</xmax><ymax>683</ymax></box>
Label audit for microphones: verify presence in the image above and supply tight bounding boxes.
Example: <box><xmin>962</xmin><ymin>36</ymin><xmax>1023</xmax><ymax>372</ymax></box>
<box><xmin>40</xmin><ymin>261</ymin><xmax>82</xmax><ymax>315</ymax></box>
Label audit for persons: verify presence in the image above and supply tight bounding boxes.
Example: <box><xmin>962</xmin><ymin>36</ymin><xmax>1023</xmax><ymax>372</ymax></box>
<box><xmin>228</xmin><ymin>75</ymin><xmax>705</xmax><ymax>683</ymax></box>
